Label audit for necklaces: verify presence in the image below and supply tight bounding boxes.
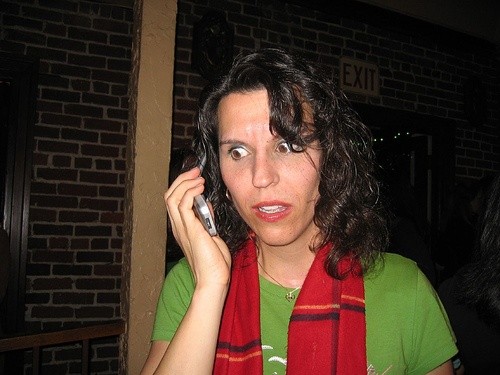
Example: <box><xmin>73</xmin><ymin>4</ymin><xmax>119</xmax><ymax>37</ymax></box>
<box><xmin>257</xmin><ymin>259</ymin><xmax>304</xmax><ymax>302</ymax></box>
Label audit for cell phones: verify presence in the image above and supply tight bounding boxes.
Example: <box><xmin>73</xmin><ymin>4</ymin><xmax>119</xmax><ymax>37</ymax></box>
<box><xmin>193</xmin><ymin>195</ymin><xmax>217</xmax><ymax>236</ymax></box>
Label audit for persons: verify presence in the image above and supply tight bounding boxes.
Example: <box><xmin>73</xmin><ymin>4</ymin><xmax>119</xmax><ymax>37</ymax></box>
<box><xmin>140</xmin><ymin>45</ymin><xmax>460</xmax><ymax>375</ymax></box>
